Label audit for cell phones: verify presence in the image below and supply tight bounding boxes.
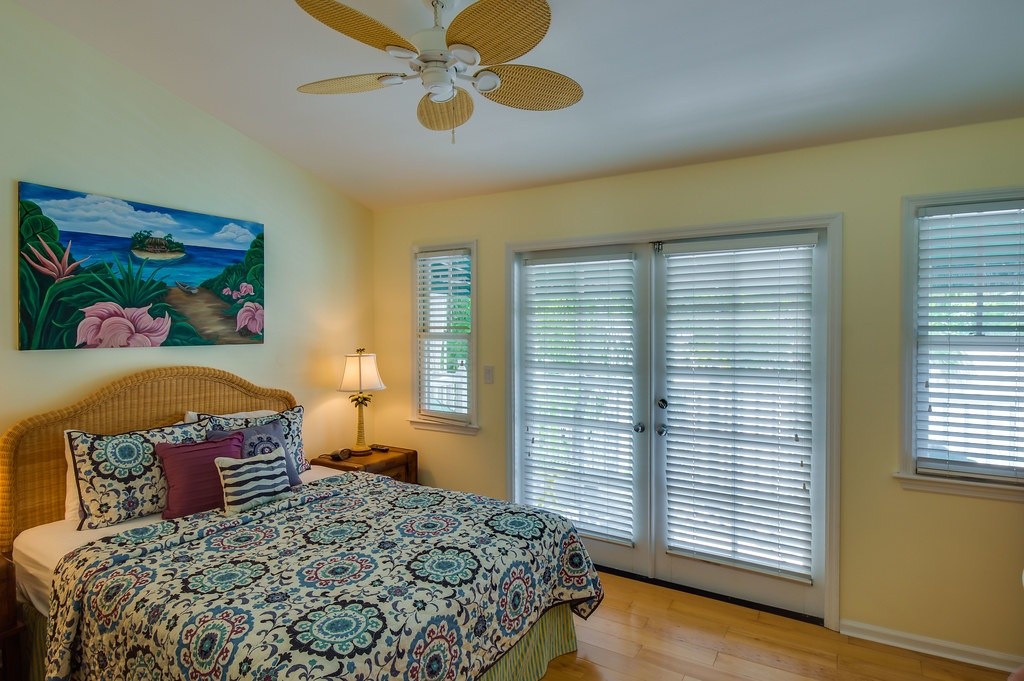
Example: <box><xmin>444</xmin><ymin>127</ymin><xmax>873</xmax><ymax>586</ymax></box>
<box><xmin>371</xmin><ymin>447</ymin><xmax>389</xmax><ymax>452</ymax></box>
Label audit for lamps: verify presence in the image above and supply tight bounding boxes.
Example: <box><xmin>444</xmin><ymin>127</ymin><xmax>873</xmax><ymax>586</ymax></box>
<box><xmin>377</xmin><ymin>1</ymin><xmax>501</xmax><ymax>145</ymax></box>
<box><xmin>338</xmin><ymin>347</ymin><xmax>386</xmax><ymax>456</ymax></box>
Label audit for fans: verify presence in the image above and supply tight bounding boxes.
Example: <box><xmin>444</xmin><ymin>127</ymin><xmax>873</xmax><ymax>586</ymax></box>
<box><xmin>294</xmin><ymin>1</ymin><xmax>583</xmax><ymax>131</ymax></box>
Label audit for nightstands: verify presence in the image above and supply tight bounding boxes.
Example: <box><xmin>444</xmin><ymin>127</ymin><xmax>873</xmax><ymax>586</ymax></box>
<box><xmin>310</xmin><ymin>444</ymin><xmax>418</xmax><ymax>485</ymax></box>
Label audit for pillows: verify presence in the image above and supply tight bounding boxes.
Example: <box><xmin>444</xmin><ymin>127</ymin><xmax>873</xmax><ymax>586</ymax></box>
<box><xmin>184</xmin><ymin>409</ymin><xmax>279</xmax><ymax>423</ymax></box>
<box><xmin>64</xmin><ymin>421</ymin><xmax>185</xmax><ymax>532</ymax></box>
<box><xmin>207</xmin><ymin>420</ymin><xmax>302</xmax><ymax>489</ymax></box>
<box><xmin>67</xmin><ymin>417</ymin><xmax>211</xmax><ymax>532</ymax></box>
<box><xmin>157</xmin><ymin>432</ymin><xmax>245</xmax><ymax>518</ymax></box>
<box><xmin>213</xmin><ymin>447</ymin><xmax>295</xmax><ymax>514</ymax></box>
<box><xmin>198</xmin><ymin>405</ymin><xmax>311</xmax><ymax>477</ymax></box>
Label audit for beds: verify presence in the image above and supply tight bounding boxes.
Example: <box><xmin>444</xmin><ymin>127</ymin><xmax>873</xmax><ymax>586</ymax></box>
<box><xmin>1</xmin><ymin>364</ymin><xmax>606</xmax><ymax>680</ymax></box>
